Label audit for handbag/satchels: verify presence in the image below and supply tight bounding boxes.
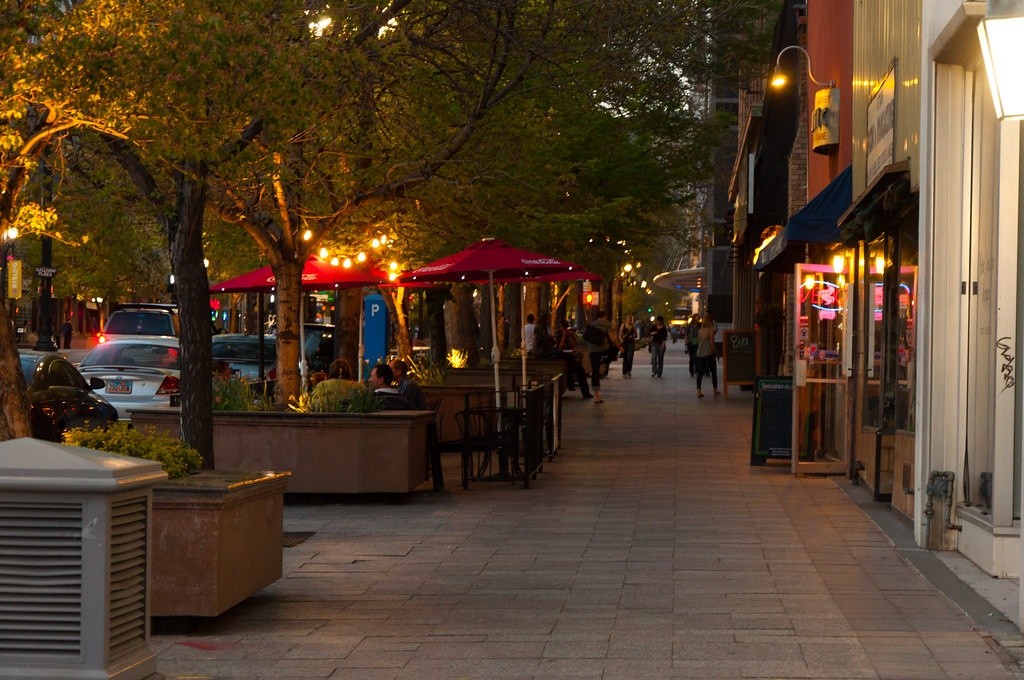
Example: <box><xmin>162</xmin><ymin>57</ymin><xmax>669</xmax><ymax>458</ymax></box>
<box><xmin>582</xmin><ymin>323</ymin><xmax>606</xmax><ymax>347</ymax></box>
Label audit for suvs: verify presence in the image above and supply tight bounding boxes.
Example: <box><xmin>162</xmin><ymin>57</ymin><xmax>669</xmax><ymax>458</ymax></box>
<box><xmin>98</xmin><ymin>305</ymin><xmax>179</xmax><ymax>344</ymax></box>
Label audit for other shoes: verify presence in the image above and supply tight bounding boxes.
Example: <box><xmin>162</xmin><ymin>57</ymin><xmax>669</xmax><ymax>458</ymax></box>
<box><xmin>697</xmin><ymin>394</ymin><xmax>705</xmax><ymax>397</ymax></box>
<box><xmin>651</xmin><ymin>371</ymin><xmax>662</xmax><ymax>377</ymax></box>
<box><xmin>600</xmin><ymin>369</ymin><xmax>604</xmax><ymax>379</ymax></box>
<box><xmin>713</xmin><ymin>390</ymin><xmax>720</xmax><ymax>396</ymax></box>
<box><xmin>593</xmin><ymin>400</ymin><xmax>604</xmax><ymax>403</ymax></box>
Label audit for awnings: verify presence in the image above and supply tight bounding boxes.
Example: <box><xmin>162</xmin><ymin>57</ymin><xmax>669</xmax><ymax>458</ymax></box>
<box><xmin>750</xmin><ymin>161</ymin><xmax>853</xmax><ymax>271</ymax></box>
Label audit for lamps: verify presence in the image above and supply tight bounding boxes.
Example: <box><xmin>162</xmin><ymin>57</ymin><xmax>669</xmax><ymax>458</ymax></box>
<box><xmin>772</xmin><ymin>46</ymin><xmax>836</xmax><ymax>88</ymax></box>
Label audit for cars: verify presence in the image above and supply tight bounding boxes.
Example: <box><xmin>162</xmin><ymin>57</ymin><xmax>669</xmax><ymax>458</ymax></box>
<box><xmin>16</xmin><ymin>334</ymin><xmax>336</xmax><ymax>432</ymax></box>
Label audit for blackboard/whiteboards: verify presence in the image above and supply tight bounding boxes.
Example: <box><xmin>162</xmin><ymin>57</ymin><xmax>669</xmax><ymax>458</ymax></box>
<box><xmin>722</xmin><ymin>329</ymin><xmax>761</xmax><ymax>385</ymax></box>
<box><xmin>750</xmin><ymin>375</ymin><xmax>814</xmax><ymax>459</ymax></box>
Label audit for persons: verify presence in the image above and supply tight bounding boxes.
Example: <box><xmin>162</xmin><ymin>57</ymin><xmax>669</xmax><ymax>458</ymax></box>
<box><xmin>212</xmin><ymin>358</ymin><xmax>231</xmax><ymax>381</ymax></box>
<box><xmin>532</xmin><ymin>312</ymin><xmax>589</xmax><ymax>397</ymax></box>
<box><xmin>327</xmin><ymin>358</ymin><xmax>353</xmax><ymax>380</ymax></box>
<box><xmin>587</xmin><ymin>312</ymin><xmax>625</xmax><ymax>405</ymax></box>
<box><xmin>60</xmin><ymin>317</ymin><xmax>74</xmax><ymax>349</ymax></box>
<box><xmin>388</xmin><ymin>359</ymin><xmax>426</xmax><ymax>411</ymax></box>
<box><xmin>368</xmin><ymin>363</ymin><xmax>411</xmax><ymax>411</ymax></box>
<box><xmin>555</xmin><ymin>317</ymin><xmax>576</xmax><ymax>352</ymax></box>
<box><xmin>523</xmin><ymin>313</ymin><xmax>536</xmax><ymax>354</ymax></box>
<box><xmin>648</xmin><ymin>315</ymin><xmax>668</xmax><ymax>378</ymax></box>
<box><xmin>619</xmin><ymin>309</ymin><xmax>722</xmax><ymax>397</ymax></box>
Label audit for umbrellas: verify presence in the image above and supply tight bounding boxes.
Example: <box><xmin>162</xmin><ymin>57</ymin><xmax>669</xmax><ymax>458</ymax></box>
<box><xmin>378</xmin><ymin>238</ymin><xmax>603</xmax><ymax>433</ymax></box>
<box><xmin>208</xmin><ymin>254</ymin><xmax>392</xmax><ymax>393</ymax></box>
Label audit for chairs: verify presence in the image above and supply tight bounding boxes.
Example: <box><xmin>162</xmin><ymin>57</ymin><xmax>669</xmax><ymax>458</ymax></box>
<box><xmin>454</xmin><ymin>384</ymin><xmax>538</xmax><ymax>480</ymax></box>
<box><xmin>373</xmin><ymin>392</ymin><xmax>444</xmax><ymax>489</ymax></box>
